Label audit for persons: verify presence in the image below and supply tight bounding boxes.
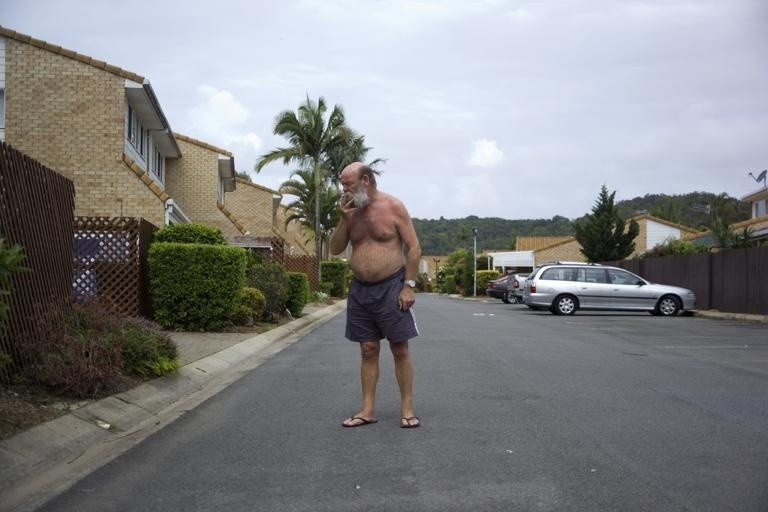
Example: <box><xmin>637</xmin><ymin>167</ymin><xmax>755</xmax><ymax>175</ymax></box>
<box><xmin>328</xmin><ymin>161</ymin><xmax>425</xmax><ymax>429</ymax></box>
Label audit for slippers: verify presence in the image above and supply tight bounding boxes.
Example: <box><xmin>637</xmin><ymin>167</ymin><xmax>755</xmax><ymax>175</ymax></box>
<box><xmin>400</xmin><ymin>416</ymin><xmax>420</xmax><ymax>428</ymax></box>
<box><xmin>343</xmin><ymin>415</ymin><xmax>378</xmax><ymax>427</ymax></box>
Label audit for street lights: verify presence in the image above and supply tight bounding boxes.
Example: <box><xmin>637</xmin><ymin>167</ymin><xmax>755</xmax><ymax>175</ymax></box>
<box><xmin>472</xmin><ymin>226</ymin><xmax>479</xmax><ymax>296</ymax></box>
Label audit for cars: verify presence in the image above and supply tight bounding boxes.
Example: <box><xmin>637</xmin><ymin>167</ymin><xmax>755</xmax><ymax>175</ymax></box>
<box><xmin>485</xmin><ymin>261</ymin><xmax>697</xmax><ymax>316</ymax></box>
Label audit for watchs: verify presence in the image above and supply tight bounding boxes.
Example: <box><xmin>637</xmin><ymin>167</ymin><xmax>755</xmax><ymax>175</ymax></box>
<box><xmin>404</xmin><ymin>279</ymin><xmax>417</xmax><ymax>288</ymax></box>
